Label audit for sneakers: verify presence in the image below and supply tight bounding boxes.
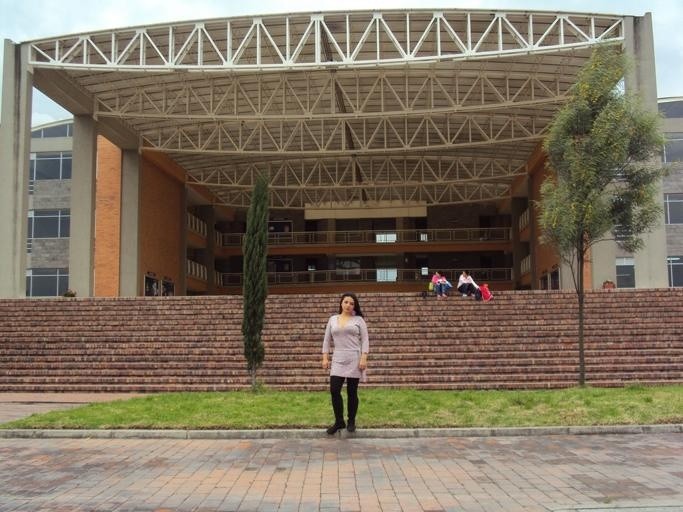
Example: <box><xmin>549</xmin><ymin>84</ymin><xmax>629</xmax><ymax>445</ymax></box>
<box><xmin>463</xmin><ymin>294</ymin><xmax>475</xmax><ymax>297</ymax></box>
<box><xmin>347</xmin><ymin>421</ymin><xmax>355</xmax><ymax>431</ymax></box>
<box><xmin>437</xmin><ymin>293</ymin><xmax>447</xmax><ymax>297</ymax></box>
<box><xmin>327</xmin><ymin>422</ymin><xmax>346</xmax><ymax>433</ymax></box>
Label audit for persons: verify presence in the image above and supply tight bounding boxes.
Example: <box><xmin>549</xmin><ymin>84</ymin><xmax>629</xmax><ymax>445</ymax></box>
<box><xmin>430</xmin><ymin>270</ymin><xmax>449</xmax><ymax>301</ymax></box>
<box><xmin>146</xmin><ymin>280</ymin><xmax>158</xmax><ymax>296</ymax></box>
<box><xmin>161</xmin><ymin>282</ymin><xmax>166</xmax><ymax>296</ymax></box>
<box><xmin>318</xmin><ymin>292</ymin><xmax>370</xmax><ymax>437</ymax></box>
<box><xmin>456</xmin><ymin>269</ymin><xmax>479</xmax><ymax>298</ymax></box>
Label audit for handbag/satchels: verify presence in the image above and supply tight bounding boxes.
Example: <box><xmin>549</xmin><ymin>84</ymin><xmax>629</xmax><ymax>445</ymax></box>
<box><xmin>475</xmin><ymin>288</ymin><xmax>481</xmax><ymax>299</ymax></box>
<box><xmin>428</xmin><ymin>282</ymin><xmax>434</xmax><ymax>291</ymax></box>
